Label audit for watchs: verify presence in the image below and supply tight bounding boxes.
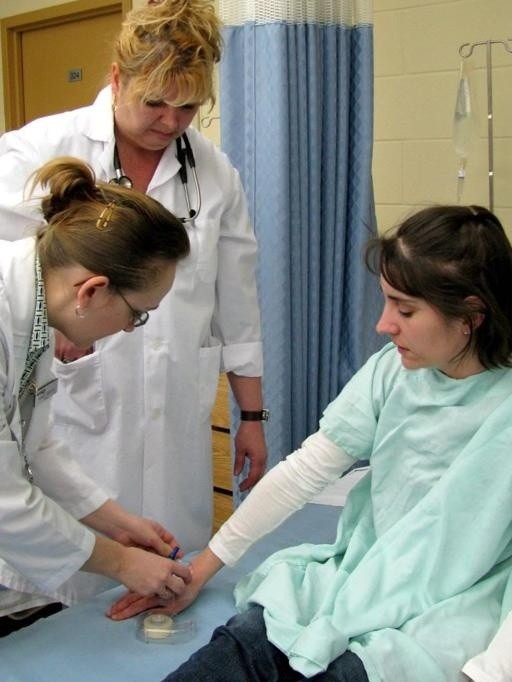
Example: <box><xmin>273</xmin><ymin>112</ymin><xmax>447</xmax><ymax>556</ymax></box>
<box><xmin>241</xmin><ymin>409</ymin><xmax>269</xmax><ymax>421</ymax></box>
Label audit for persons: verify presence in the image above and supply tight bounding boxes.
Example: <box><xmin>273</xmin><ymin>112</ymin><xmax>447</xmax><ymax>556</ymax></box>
<box><xmin>0</xmin><ymin>158</ymin><xmax>192</xmax><ymax>601</ymax></box>
<box><xmin>0</xmin><ymin>0</ymin><xmax>270</xmax><ymax>609</ymax></box>
<box><xmin>105</xmin><ymin>205</ymin><xmax>512</xmax><ymax>682</ymax></box>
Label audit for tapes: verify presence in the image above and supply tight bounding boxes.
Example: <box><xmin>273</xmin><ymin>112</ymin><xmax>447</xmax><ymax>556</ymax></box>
<box><xmin>142</xmin><ymin>614</ymin><xmax>173</xmax><ymax>639</ymax></box>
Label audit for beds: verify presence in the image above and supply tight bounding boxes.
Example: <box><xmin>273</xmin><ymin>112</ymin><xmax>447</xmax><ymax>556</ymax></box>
<box><xmin>0</xmin><ymin>466</ymin><xmax>372</xmax><ymax>679</ymax></box>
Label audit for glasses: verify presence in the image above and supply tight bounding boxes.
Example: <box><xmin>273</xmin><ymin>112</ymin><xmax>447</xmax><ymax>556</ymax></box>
<box><xmin>73</xmin><ymin>275</ymin><xmax>149</xmax><ymax>328</ymax></box>
